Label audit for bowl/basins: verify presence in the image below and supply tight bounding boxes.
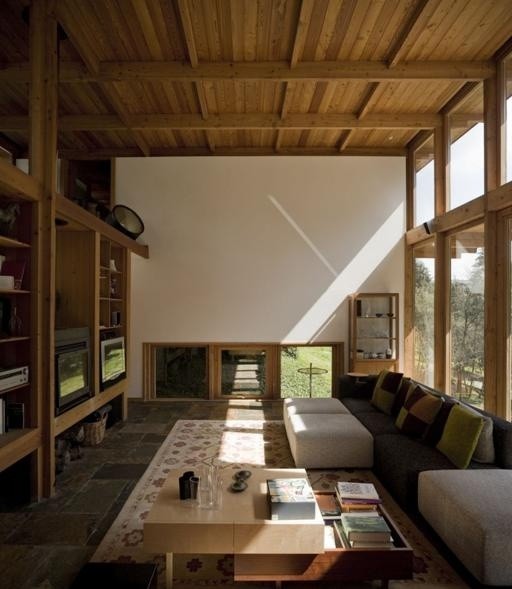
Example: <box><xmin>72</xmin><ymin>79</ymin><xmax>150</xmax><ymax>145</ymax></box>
<box><xmin>376</xmin><ymin>313</ymin><xmax>394</xmax><ymax>318</ymax></box>
<box><xmin>364</xmin><ymin>348</ymin><xmax>393</xmax><ymax>360</ymax></box>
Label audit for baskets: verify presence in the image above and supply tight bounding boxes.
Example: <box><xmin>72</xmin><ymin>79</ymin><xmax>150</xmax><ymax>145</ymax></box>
<box><xmin>83</xmin><ymin>411</ymin><xmax>108</xmax><ymax>446</ymax></box>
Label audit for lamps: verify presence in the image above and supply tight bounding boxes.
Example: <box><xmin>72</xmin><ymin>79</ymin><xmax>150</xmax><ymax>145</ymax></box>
<box><xmin>106</xmin><ymin>205</ymin><xmax>146</xmax><ymax>240</ymax></box>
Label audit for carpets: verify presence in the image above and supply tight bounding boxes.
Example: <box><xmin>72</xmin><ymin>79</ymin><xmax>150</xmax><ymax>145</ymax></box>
<box><xmin>92</xmin><ymin>418</ymin><xmax>465</xmax><ymax>588</ymax></box>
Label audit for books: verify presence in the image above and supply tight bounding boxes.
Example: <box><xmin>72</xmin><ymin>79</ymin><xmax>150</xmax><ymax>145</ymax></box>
<box><xmin>265</xmin><ymin>476</ymin><xmax>395</xmax><ymax>549</ymax></box>
<box><xmin>0</xmin><ymin>256</ymin><xmax>28</xmax><ymax>290</ymax></box>
<box><xmin>0</xmin><ymin>397</ymin><xmax>25</xmax><ymax>437</ymax></box>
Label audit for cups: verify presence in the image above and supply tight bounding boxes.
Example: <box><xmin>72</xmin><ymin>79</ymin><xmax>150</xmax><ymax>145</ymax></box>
<box><xmin>190</xmin><ymin>477</ymin><xmax>207</xmax><ymax>508</ymax></box>
<box><xmin>14</xmin><ymin>280</ymin><xmax>22</xmax><ymax>289</ymax></box>
<box><xmin>16</xmin><ymin>158</ymin><xmax>29</xmax><ymax>174</ymax></box>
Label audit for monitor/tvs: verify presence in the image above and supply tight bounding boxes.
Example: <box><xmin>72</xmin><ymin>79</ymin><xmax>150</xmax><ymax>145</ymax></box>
<box><xmin>101</xmin><ymin>335</ymin><xmax>125</xmax><ymax>387</ymax></box>
<box><xmin>54</xmin><ymin>327</ymin><xmax>91</xmax><ymax>415</ymax></box>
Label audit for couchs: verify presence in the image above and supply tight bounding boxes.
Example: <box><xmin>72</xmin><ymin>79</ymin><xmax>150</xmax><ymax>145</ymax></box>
<box><xmin>338</xmin><ymin>374</ymin><xmax>512</xmax><ymax>589</ymax></box>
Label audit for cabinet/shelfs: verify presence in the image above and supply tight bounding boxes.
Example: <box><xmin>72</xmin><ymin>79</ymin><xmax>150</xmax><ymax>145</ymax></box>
<box><xmin>347</xmin><ymin>293</ymin><xmax>400</xmax><ymax>375</ymax></box>
<box><xmin>232</xmin><ymin>489</ymin><xmax>414</xmax><ymax>588</ymax></box>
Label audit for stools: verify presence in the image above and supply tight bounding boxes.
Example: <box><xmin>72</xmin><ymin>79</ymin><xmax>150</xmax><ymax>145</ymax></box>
<box><xmin>282</xmin><ymin>399</ymin><xmax>373</xmax><ymax>469</ymax></box>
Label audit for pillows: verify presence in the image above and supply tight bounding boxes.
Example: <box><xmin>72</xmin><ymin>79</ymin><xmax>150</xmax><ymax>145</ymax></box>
<box><xmin>369</xmin><ymin>370</ymin><xmax>496</xmax><ymax>469</ymax></box>
